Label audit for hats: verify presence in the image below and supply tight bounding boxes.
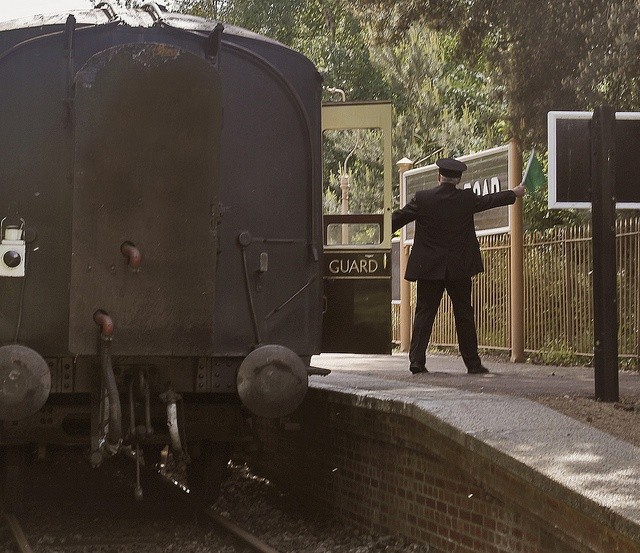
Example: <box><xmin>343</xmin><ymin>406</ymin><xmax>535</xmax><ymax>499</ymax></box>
<box><xmin>436</xmin><ymin>158</ymin><xmax>468</xmax><ymax>177</ymax></box>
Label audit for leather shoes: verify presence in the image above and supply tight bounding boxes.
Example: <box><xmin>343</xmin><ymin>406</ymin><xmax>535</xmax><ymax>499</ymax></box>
<box><xmin>469</xmin><ymin>364</ymin><xmax>489</xmax><ymax>374</ymax></box>
<box><xmin>409</xmin><ymin>362</ymin><xmax>428</xmax><ymax>375</ymax></box>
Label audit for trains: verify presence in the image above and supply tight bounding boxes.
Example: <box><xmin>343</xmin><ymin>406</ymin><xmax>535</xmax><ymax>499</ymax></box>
<box><xmin>0</xmin><ymin>2</ymin><xmax>393</xmax><ymax>508</ymax></box>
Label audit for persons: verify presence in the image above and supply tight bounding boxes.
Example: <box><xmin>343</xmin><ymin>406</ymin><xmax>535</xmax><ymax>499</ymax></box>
<box><xmin>372</xmin><ymin>156</ymin><xmax>526</xmax><ymax>375</ymax></box>
